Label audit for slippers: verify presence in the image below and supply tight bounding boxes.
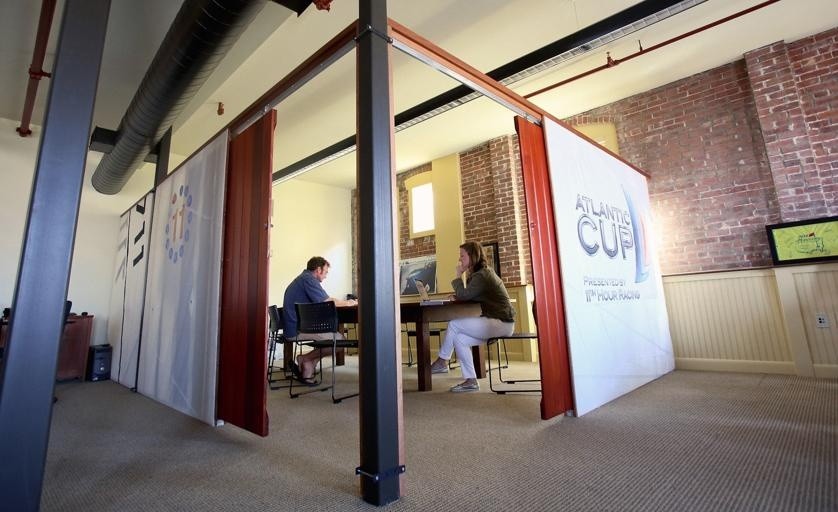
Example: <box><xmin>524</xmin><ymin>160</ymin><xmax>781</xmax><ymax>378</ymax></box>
<box><xmin>289</xmin><ymin>358</ymin><xmax>318</xmax><ymax>385</ymax></box>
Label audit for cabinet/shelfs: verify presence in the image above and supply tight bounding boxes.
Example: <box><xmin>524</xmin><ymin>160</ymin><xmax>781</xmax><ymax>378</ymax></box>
<box><xmin>0</xmin><ymin>315</ymin><xmax>94</xmax><ymax>383</ymax></box>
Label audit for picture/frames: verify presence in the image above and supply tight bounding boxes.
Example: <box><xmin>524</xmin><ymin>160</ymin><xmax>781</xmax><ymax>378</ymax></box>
<box><xmin>482</xmin><ymin>242</ymin><xmax>500</xmax><ymax>279</ymax></box>
<box><xmin>399</xmin><ymin>252</ymin><xmax>437</xmax><ymax>296</ymax></box>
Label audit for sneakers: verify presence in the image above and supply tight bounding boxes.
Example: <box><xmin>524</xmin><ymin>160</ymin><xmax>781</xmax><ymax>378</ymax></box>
<box><xmin>449</xmin><ymin>376</ymin><xmax>479</xmax><ymax>393</ymax></box>
<box><xmin>431</xmin><ymin>358</ymin><xmax>450</xmax><ymax>374</ymax></box>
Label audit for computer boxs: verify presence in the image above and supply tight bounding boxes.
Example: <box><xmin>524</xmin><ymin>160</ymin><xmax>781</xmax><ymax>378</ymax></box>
<box><xmin>85</xmin><ymin>344</ymin><xmax>112</xmax><ymax>382</ymax></box>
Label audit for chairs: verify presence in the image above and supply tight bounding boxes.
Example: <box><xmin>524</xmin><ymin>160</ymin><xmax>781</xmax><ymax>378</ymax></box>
<box><xmin>400</xmin><ymin>322</ymin><xmax>461</xmax><ymax>371</ymax></box>
<box><xmin>487</xmin><ymin>298</ymin><xmax>543</xmax><ymax>394</ymax></box>
<box><xmin>338</xmin><ymin>294</ymin><xmax>357</xmax><ymax>355</ymax></box>
<box><xmin>263</xmin><ymin>302</ymin><xmax>360</xmax><ymax>402</ymax></box>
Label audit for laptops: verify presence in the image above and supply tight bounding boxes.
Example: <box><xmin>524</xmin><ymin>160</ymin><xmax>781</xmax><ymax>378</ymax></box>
<box><xmin>414</xmin><ymin>279</ymin><xmax>447</xmax><ymax>301</ymax></box>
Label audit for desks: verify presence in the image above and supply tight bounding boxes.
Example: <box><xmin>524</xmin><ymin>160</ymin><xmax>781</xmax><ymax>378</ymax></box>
<box><xmin>327</xmin><ymin>299</ymin><xmax>487</xmax><ymax>392</ymax></box>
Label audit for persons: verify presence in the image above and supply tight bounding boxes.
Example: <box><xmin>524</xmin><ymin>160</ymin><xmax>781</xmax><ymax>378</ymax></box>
<box><xmin>279</xmin><ymin>255</ymin><xmax>358</xmax><ymax>388</ymax></box>
<box><xmin>432</xmin><ymin>242</ymin><xmax>518</xmax><ymax>393</ymax></box>
